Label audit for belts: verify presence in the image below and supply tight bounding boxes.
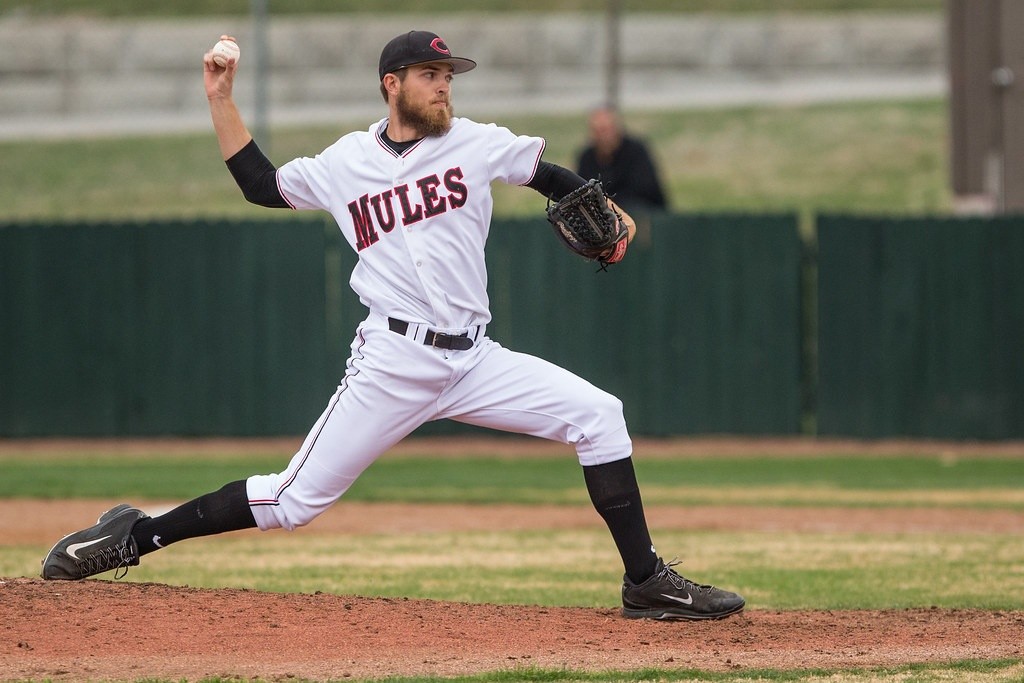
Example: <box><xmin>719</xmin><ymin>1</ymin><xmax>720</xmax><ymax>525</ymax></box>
<box><xmin>388</xmin><ymin>317</ymin><xmax>480</xmax><ymax>350</ymax></box>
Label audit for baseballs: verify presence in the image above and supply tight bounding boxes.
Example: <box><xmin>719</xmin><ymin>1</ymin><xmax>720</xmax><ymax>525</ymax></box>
<box><xmin>213</xmin><ymin>40</ymin><xmax>241</xmax><ymax>67</ymax></box>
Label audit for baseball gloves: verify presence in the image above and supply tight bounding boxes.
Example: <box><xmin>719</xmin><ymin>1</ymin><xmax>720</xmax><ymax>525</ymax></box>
<box><xmin>546</xmin><ymin>179</ymin><xmax>628</xmax><ymax>263</ymax></box>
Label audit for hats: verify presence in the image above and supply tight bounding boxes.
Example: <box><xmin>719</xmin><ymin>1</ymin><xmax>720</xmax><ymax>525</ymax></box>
<box><xmin>379</xmin><ymin>30</ymin><xmax>476</xmax><ymax>82</ymax></box>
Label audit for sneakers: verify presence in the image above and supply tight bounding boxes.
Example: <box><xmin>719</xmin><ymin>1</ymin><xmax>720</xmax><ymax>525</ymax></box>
<box><xmin>40</xmin><ymin>503</ymin><xmax>152</xmax><ymax>580</ymax></box>
<box><xmin>622</xmin><ymin>556</ymin><xmax>745</xmax><ymax>619</ymax></box>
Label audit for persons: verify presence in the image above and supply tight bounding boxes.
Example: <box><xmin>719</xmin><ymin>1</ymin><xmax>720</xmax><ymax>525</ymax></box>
<box><xmin>568</xmin><ymin>105</ymin><xmax>679</xmax><ymax>216</ymax></box>
<box><xmin>38</xmin><ymin>29</ymin><xmax>746</xmax><ymax>621</ymax></box>
